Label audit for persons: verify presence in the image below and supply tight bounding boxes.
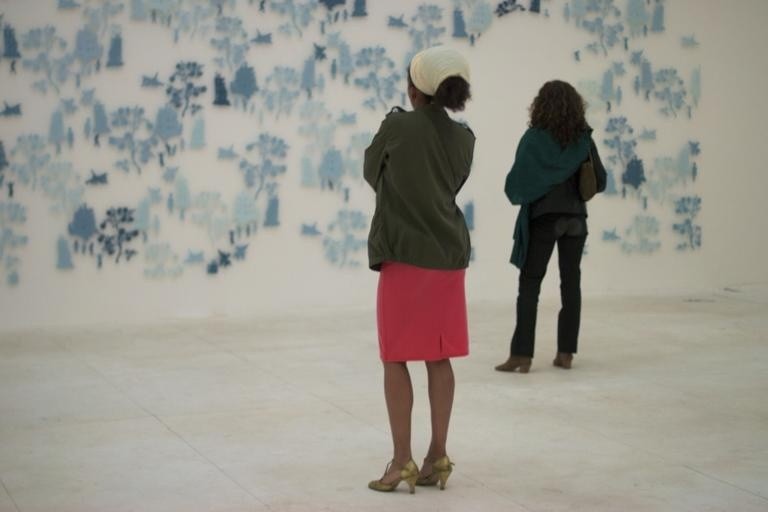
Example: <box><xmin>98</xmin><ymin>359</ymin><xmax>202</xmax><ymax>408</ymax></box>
<box><xmin>493</xmin><ymin>80</ymin><xmax>607</xmax><ymax>372</ymax></box>
<box><xmin>362</xmin><ymin>44</ymin><xmax>475</xmax><ymax>493</ymax></box>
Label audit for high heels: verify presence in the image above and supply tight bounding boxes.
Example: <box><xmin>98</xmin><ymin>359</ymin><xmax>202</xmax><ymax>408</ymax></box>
<box><xmin>416</xmin><ymin>455</ymin><xmax>455</xmax><ymax>490</ymax></box>
<box><xmin>368</xmin><ymin>458</ymin><xmax>419</xmax><ymax>493</ymax></box>
<box><xmin>553</xmin><ymin>352</ymin><xmax>572</xmax><ymax>368</ymax></box>
<box><xmin>495</xmin><ymin>357</ymin><xmax>531</xmax><ymax>373</ymax></box>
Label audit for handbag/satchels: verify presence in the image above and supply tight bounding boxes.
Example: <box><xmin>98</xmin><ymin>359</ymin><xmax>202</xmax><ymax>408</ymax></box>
<box><xmin>580</xmin><ymin>161</ymin><xmax>596</xmax><ymax>200</ymax></box>
